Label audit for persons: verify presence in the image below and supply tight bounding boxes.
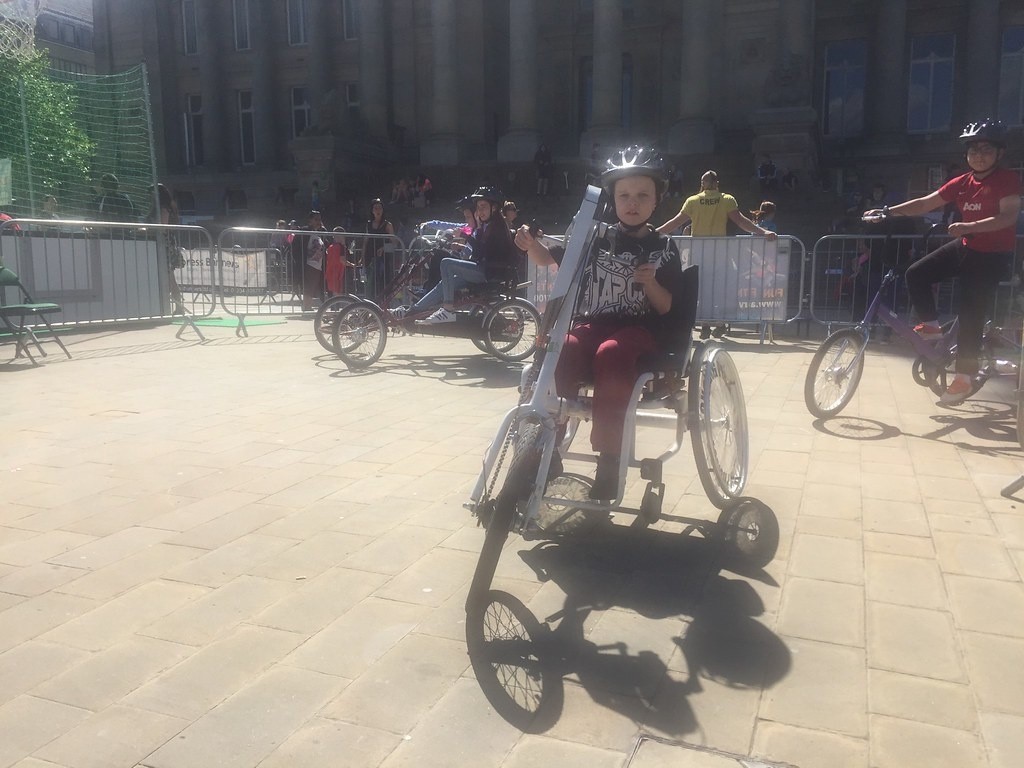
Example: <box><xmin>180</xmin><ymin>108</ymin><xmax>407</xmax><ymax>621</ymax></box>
<box><xmin>143</xmin><ymin>182</ymin><xmax>184</xmax><ymax>315</ymax></box>
<box><xmin>654</xmin><ymin>170</ymin><xmax>778</xmax><ymax>339</ymax></box>
<box><xmin>387</xmin><ymin>176</ymin><xmax>432</xmax><ymax>206</ymax></box>
<box><xmin>94</xmin><ymin>173</ymin><xmax>137</xmax><ymax>240</ymax></box>
<box><xmin>757</xmin><ymin>154</ymin><xmax>780</xmax><ymax>194</ymax></box>
<box><xmin>311</xmin><ymin>180</ymin><xmax>321</xmax><ymax>208</ymax></box>
<box><xmin>666</xmin><ymin>164</ymin><xmax>682</xmax><ymax>198</ymax></box>
<box><xmin>514</xmin><ymin>144</ymin><xmax>688</xmax><ymax>501</ymax></box>
<box><xmin>385</xmin><ymin>184</ymin><xmax>516</xmax><ymax>324</ymax></box>
<box><xmin>862</xmin><ymin>118</ymin><xmax>1021</xmax><ymax>404</ymax></box>
<box><xmin>830</xmin><ymin>162</ymin><xmax>989</xmax><ymax>344</ymax></box>
<box><xmin>503</xmin><ymin>202</ymin><xmax>520</xmax><ymax>230</ymax></box>
<box><xmin>344</xmin><ymin>199</ymin><xmax>357</xmax><ymax>227</ymax></box>
<box><xmin>584</xmin><ymin>142</ymin><xmax>604</xmax><ymax>188</ymax></box>
<box><xmin>750</xmin><ymin>201</ymin><xmax>777</xmax><ymax>236</ymax></box>
<box><xmin>535</xmin><ymin>144</ymin><xmax>566</xmax><ymax>204</ymax></box>
<box><xmin>36</xmin><ymin>194</ymin><xmax>61</xmax><ymax>238</ymax></box>
<box><xmin>271</xmin><ymin>210</ymin><xmax>361</xmax><ymax>311</ymax></box>
<box><xmin>361</xmin><ymin>198</ymin><xmax>402</xmax><ymax>302</ymax></box>
<box><xmin>682</xmin><ymin>221</ymin><xmax>693</xmax><ymax>235</ymax></box>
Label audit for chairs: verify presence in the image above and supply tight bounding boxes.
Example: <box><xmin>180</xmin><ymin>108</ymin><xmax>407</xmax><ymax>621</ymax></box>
<box><xmin>0</xmin><ymin>265</ymin><xmax>72</xmax><ymax>367</ymax></box>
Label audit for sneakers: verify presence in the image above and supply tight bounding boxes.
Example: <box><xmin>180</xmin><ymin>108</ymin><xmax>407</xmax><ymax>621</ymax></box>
<box><xmin>589</xmin><ymin>455</ymin><xmax>621</xmax><ymax>501</ymax></box>
<box><xmin>390</xmin><ymin>306</ymin><xmax>406</xmax><ymax>318</ymax></box>
<box><xmin>526</xmin><ymin>451</ymin><xmax>564</xmax><ymax>498</ymax></box>
<box><xmin>939</xmin><ymin>376</ymin><xmax>972</xmax><ymax>404</ymax></box>
<box><xmin>414</xmin><ymin>308</ymin><xmax>456</xmax><ymax>323</ymax></box>
<box><xmin>911</xmin><ymin>324</ymin><xmax>944</xmax><ymax>339</ymax></box>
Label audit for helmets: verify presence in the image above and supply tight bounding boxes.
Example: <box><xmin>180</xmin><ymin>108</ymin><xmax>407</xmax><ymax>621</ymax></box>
<box><xmin>469</xmin><ymin>187</ymin><xmax>505</xmax><ymax>205</ymax></box>
<box><xmin>600</xmin><ymin>145</ymin><xmax>671</xmax><ymax>198</ymax></box>
<box><xmin>958</xmin><ymin>116</ymin><xmax>1009</xmax><ymax>143</ymax></box>
<box><xmin>456</xmin><ymin>196</ymin><xmax>477</xmax><ymax>211</ymax></box>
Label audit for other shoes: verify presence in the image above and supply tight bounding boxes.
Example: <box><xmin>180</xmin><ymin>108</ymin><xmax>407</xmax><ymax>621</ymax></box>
<box><xmin>881</xmin><ymin>338</ymin><xmax>890</xmax><ymax>345</ymax></box>
<box><xmin>173</xmin><ymin>311</ymin><xmax>185</xmax><ymax>316</ymax></box>
<box><xmin>712</xmin><ymin>325</ymin><xmax>727</xmax><ymax>337</ymax></box>
<box><xmin>867</xmin><ymin>333</ymin><xmax>876</xmax><ymax>342</ymax></box>
<box><xmin>700</xmin><ymin>329</ymin><xmax>711</xmax><ymax>339</ymax></box>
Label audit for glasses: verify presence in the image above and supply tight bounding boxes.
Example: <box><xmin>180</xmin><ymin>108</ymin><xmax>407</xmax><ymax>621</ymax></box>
<box><xmin>969</xmin><ymin>145</ymin><xmax>999</xmax><ymax>154</ymax></box>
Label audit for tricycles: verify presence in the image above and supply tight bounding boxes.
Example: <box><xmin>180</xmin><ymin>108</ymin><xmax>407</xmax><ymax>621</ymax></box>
<box><xmin>804</xmin><ymin>202</ymin><xmax>1024</xmax><ymax>442</ymax></box>
<box><xmin>311</xmin><ymin>220</ymin><xmax>544</xmax><ymax>370</ymax></box>
<box><xmin>463</xmin><ymin>183</ymin><xmax>751</xmax><ymax>620</ymax></box>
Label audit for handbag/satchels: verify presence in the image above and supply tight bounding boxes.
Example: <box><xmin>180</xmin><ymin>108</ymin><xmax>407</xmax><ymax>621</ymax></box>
<box><xmin>173</xmin><ymin>247</ymin><xmax>188</xmax><ymax>268</ymax></box>
